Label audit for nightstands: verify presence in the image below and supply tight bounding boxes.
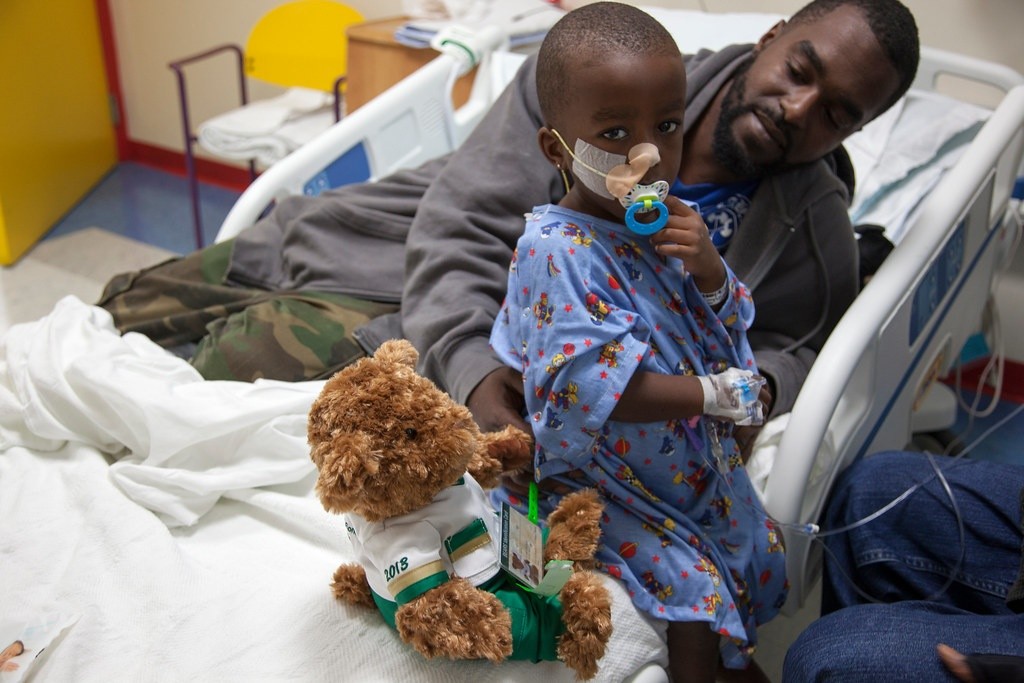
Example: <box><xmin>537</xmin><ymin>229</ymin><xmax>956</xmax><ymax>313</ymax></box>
<box><xmin>345</xmin><ymin>14</ymin><xmax>480</xmax><ymax>118</ymax></box>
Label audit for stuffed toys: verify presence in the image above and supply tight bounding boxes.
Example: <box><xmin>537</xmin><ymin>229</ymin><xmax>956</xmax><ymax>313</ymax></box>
<box><xmin>308</xmin><ymin>340</ymin><xmax>610</xmax><ymax>683</ymax></box>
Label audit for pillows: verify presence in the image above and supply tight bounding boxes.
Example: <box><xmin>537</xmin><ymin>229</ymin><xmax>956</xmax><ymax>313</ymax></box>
<box><xmin>643</xmin><ymin>11</ymin><xmax>906</xmax><ymax>201</ymax></box>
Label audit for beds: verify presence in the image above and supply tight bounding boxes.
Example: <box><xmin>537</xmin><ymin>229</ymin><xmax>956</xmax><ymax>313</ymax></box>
<box><xmin>0</xmin><ymin>7</ymin><xmax>1024</xmax><ymax>683</ymax></box>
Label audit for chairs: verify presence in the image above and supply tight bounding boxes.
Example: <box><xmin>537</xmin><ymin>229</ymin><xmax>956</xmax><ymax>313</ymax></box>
<box><xmin>169</xmin><ymin>0</ymin><xmax>368</xmax><ymax>252</ymax></box>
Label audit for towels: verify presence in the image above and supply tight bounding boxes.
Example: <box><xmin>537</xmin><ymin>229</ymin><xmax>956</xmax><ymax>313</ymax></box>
<box><xmin>195</xmin><ymin>86</ymin><xmax>346</xmax><ymax>165</ymax></box>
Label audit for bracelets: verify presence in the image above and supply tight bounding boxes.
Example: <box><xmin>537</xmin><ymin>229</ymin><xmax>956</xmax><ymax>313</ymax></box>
<box><xmin>701</xmin><ymin>276</ymin><xmax>727</xmax><ymax>305</ymax></box>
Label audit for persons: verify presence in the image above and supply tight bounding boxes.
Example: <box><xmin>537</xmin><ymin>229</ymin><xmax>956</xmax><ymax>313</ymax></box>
<box><xmin>95</xmin><ymin>0</ymin><xmax>919</xmax><ymax>432</ymax></box>
<box><xmin>782</xmin><ymin>451</ymin><xmax>1024</xmax><ymax>682</ymax></box>
<box><xmin>488</xmin><ymin>2</ymin><xmax>788</xmax><ymax>683</ymax></box>
